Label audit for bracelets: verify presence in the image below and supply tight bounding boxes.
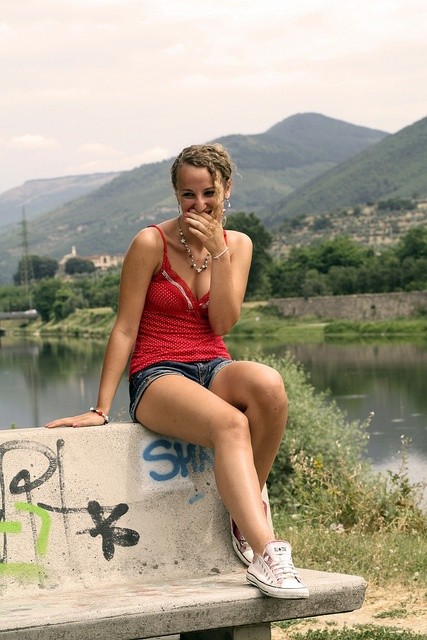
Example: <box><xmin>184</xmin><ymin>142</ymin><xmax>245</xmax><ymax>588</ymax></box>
<box><xmin>210</xmin><ymin>247</ymin><xmax>229</xmax><ymax>260</ymax></box>
<box><xmin>89</xmin><ymin>407</ymin><xmax>109</xmax><ymax>424</ymax></box>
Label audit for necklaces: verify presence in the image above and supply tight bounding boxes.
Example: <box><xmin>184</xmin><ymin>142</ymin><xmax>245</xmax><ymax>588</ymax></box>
<box><xmin>177</xmin><ymin>214</ymin><xmax>213</xmax><ymax>274</ymax></box>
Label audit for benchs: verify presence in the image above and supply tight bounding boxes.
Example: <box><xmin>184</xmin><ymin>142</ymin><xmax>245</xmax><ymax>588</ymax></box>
<box><xmin>0</xmin><ymin>422</ymin><xmax>366</xmax><ymax>640</ymax></box>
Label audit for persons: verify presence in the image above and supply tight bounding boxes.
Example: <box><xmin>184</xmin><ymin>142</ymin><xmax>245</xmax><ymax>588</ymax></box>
<box><xmin>44</xmin><ymin>143</ymin><xmax>309</xmax><ymax>601</ymax></box>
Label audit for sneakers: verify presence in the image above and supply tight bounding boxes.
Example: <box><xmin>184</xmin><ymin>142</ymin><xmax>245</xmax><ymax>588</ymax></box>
<box><xmin>246</xmin><ymin>540</ymin><xmax>310</xmax><ymax>600</ymax></box>
<box><xmin>229</xmin><ymin>500</ymin><xmax>268</xmax><ymax>568</ymax></box>
